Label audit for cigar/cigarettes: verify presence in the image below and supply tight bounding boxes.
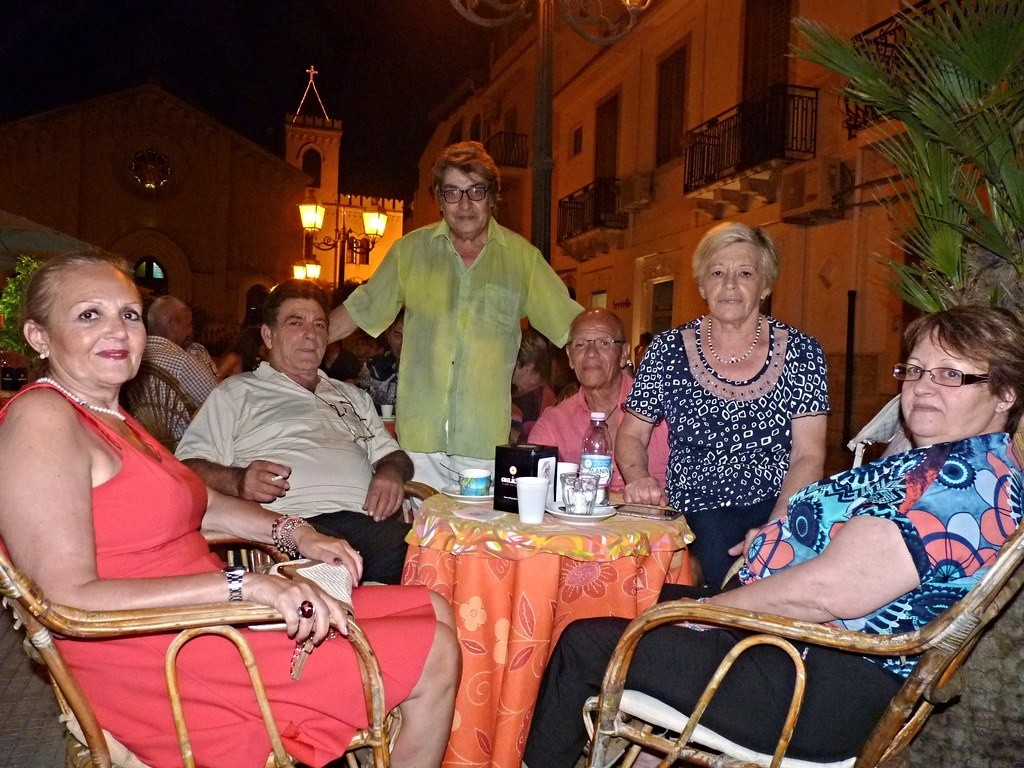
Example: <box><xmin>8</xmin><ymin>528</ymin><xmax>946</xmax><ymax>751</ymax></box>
<box><xmin>271</xmin><ymin>475</ymin><xmax>283</xmax><ymax>481</ymax></box>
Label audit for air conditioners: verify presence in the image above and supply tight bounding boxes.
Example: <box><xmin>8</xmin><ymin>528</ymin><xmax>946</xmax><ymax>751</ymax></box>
<box><xmin>621</xmin><ymin>172</ymin><xmax>652</xmax><ymax>214</ymax></box>
<box><xmin>779</xmin><ymin>158</ymin><xmax>852</xmax><ymax>226</ymax></box>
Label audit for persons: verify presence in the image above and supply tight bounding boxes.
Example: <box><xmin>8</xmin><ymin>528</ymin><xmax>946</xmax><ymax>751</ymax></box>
<box><xmin>123</xmin><ymin>283</ymin><xmax>272</xmax><ymax>452</ymax></box>
<box><xmin>529</xmin><ymin>309</ymin><xmax>669</xmax><ymax>494</ymax></box>
<box><xmin>320</xmin><ymin>280</ymin><xmax>406</xmax><ymax>414</ymax></box>
<box><xmin>635</xmin><ymin>332</ymin><xmax>653</xmax><ymax>369</ymax></box>
<box><xmin>326</xmin><ymin>140</ymin><xmax>633</xmax><ymax>488</ymax></box>
<box><xmin>0</xmin><ymin>251</ymin><xmax>464</xmax><ymax>768</ymax></box>
<box><xmin>614</xmin><ymin>222</ymin><xmax>832</xmax><ymax>592</ymax></box>
<box><xmin>509</xmin><ymin>330</ymin><xmax>579</xmax><ymax>443</ymax></box>
<box><xmin>521</xmin><ymin>306</ymin><xmax>1024</xmax><ymax>768</ymax></box>
<box><xmin>173</xmin><ymin>280</ymin><xmax>416</xmax><ymax>587</ymax></box>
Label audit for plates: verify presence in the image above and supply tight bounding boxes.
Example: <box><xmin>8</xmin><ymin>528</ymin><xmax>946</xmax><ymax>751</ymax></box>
<box><xmin>381</xmin><ymin>416</ymin><xmax>395</xmax><ymax>422</ymax></box>
<box><xmin>545</xmin><ymin>502</ymin><xmax>617</xmax><ymax>526</ymax></box>
<box><xmin>441</xmin><ymin>484</ymin><xmax>494</xmax><ymax>504</ymax></box>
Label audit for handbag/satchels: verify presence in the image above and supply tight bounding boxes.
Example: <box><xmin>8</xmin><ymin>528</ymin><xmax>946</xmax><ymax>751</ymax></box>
<box><xmin>249</xmin><ymin>560</ymin><xmax>352</xmax><ymax>632</ymax></box>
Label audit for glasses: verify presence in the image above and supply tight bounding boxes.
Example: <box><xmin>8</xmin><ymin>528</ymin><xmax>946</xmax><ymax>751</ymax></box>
<box><xmin>440</xmin><ymin>186</ymin><xmax>489</xmax><ymax>204</ymax></box>
<box><xmin>891</xmin><ymin>363</ymin><xmax>991</xmax><ymax>388</ymax></box>
<box><xmin>330</xmin><ymin>401</ymin><xmax>375</xmax><ymax>443</ymax></box>
<box><xmin>567</xmin><ymin>337</ymin><xmax>624</xmax><ymax>349</ymax></box>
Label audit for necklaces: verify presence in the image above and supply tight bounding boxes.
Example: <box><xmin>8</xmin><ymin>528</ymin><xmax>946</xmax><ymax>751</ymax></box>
<box><xmin>604</xmin><ymin>405</ymin><xmax>617</xmax><ymax>428</ymax></box>
<box><xmin>35</xmin><ymin>377</ymin><xmax>126</xmax><ymax>420</ymax></box>
<box><xmin>708</xmin><ymin>314</ymin><xmax>762</xmax><ymax>363</ymax></box>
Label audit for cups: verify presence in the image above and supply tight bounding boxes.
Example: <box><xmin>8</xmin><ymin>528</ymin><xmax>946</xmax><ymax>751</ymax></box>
<box><xmin>380</xmin><ymin>404</ymin><xmax>394</xmax><ymax>418</ymax></box>
<box><xmin>515</xmin><ymin>476</ymin><xmax>550</xmax><ymax>526</ymax></box>
<box><xmin>560</xmin><ymin>473</ymin><xmax>600</xmax><ymax>515</ymax></box>
<box><xmin>556</xmin><ymin>462</ymin><xmax>578</xmax><ymax>502</ymax></box>
<box><xmin>459</xmin><ymin>469</ymin><xmax>491</xmax><ymax>495</ymax></box>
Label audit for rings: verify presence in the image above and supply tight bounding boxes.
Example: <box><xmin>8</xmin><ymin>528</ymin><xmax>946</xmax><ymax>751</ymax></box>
<box><xmin>357</xmin><ymin>551</ymin><xmax>360</xmax><ymax>554</ymax></box>
<box><xmin>297</xmin><ymin>601</ymin><xmax>316</xmax><ymax>618</ymax></box>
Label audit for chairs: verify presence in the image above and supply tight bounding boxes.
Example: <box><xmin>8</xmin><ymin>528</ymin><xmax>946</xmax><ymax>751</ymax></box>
<box><xmin>119</xmin><ymin>360</ymin><xmax>198</xmax><ymax>455</ymax></box>
<box><xmin>584</xmin><ymin>517</ymin><xmax>1024</xmax><ymax>768</ymax></box>
<box><xmin>0</xmin><ymin>530</ymin><xmax>403</xmax><ymax>768</ymax></box>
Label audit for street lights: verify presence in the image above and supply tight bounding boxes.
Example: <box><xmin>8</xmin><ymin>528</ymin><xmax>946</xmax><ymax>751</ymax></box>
<box><xmin>294</xmin><ymin>187</ymin><xmax>389</xmax><ymax>308</ymax></box>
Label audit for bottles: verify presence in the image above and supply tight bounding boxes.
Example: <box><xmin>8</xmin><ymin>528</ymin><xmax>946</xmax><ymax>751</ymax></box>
<box><xmin>581</xmin><ymin>412</ymin><xmax>614</xmax><ymax>506</ymax></box>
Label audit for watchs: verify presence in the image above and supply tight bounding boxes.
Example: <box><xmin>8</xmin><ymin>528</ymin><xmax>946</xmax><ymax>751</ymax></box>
<box><xmin>220</xmin><ymin>566</ymin><xmax>249</xmax><ymax>602</ymax></box>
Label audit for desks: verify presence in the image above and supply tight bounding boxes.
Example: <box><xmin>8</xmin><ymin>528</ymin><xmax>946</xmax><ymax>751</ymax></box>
<box><xmin>418</xmin><ymin>494</ymin><xmax>686</xmax><ymax>768</ymax></box>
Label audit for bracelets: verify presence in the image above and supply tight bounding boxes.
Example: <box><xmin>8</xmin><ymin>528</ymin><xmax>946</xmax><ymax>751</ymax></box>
<box><xmin>272</xmin><ymin>514</ymin><xmax>316</xmax><ymax>558</ymax></box>
<box><xmin>686</xmin><ymin>597</ymin><xmax>710</xmax><ymax>631</ymax></box>
